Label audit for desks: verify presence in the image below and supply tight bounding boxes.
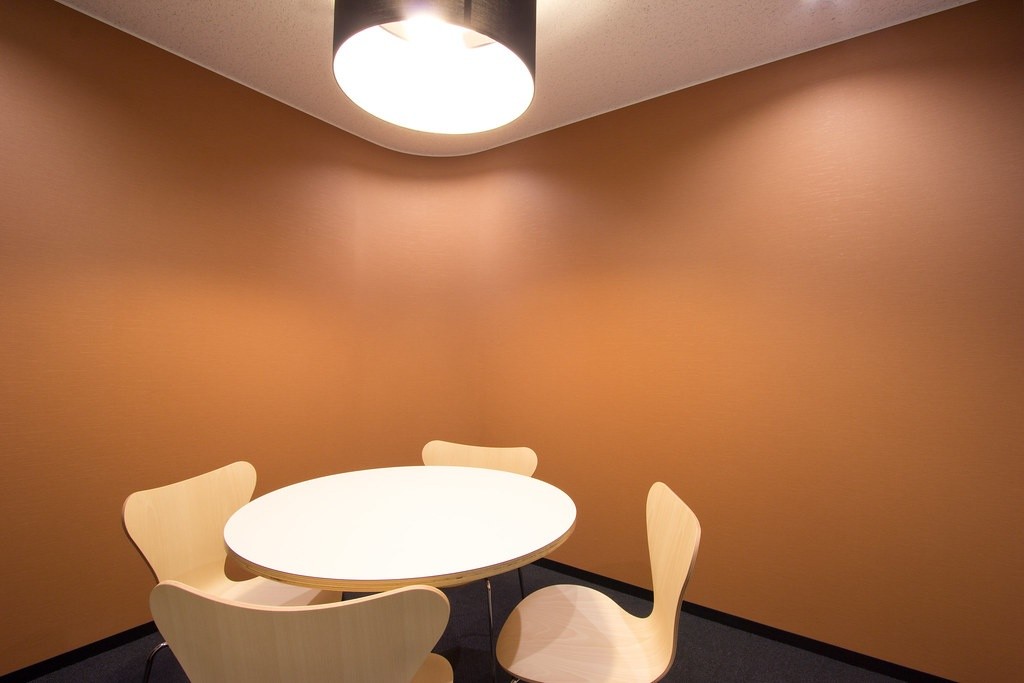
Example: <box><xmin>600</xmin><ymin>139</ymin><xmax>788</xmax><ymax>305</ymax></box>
<box><xmin>226</xmin><ymin>465</ymin><xmax>579</xmax><ymax>593</ymax></box>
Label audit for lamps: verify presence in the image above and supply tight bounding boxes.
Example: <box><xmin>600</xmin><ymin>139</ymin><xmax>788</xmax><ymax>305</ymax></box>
<box><xmin>333</xmin><ymin>0</ymin><xmax>536</xmax><ymax>134</ymax></box>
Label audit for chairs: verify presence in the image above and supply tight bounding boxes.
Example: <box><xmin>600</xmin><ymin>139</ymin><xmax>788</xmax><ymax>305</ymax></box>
<box><xmin>122</xmin><ymin>460</ymin><xmax>343</xmax><ymax>683</ymax></box>
<box><xmin>421</xmin><ymin>440</ymin><xmax>537</xmax><ymax>662</ymax></box>
<box><xmin>149</xmin><ymin>579</ymin><xmax>454</xmax><ymax>683</ymax></box>
<box><xmin>494</xmin><ymin>482</ymin><xmax>702</xmax><ymax>683</ymax></box>
